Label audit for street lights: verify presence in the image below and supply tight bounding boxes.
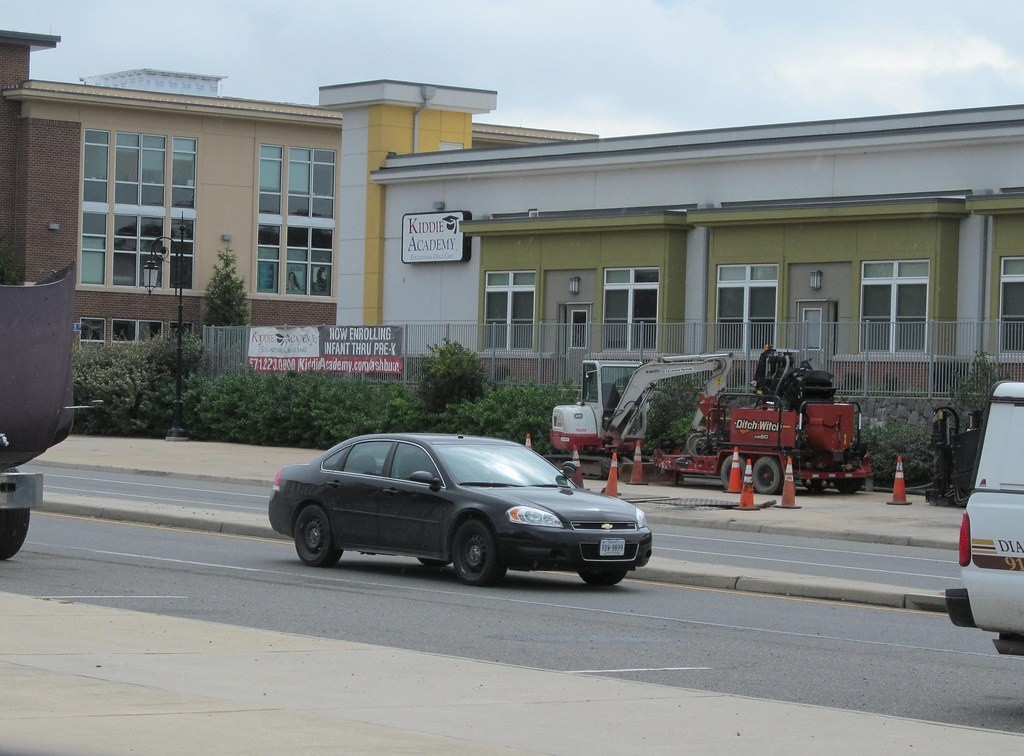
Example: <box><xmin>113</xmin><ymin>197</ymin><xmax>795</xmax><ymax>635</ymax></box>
<box><xmin>142</xmin><ymin>209</ymin><xmax>191</xmax><ymax>440</ymax></box>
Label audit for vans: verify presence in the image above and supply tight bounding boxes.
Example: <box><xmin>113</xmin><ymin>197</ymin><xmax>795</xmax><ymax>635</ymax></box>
<box><xmin>945</xmin><ymin>379</ymin><xmax>1024</xmax><ymax>657</ymax></box>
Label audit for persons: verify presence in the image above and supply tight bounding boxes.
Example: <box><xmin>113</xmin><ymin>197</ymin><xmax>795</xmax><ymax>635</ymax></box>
<box><xmin>601</xmin><ymin>372</ymin><xmax>640</xmax><ymax>431</ymax></box>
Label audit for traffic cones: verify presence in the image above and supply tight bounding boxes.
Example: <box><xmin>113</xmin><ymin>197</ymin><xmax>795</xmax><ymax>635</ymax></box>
<box><xmin>571</xmin><ymin>444</ymin><xmax>590</xmax><ymax>490</ymax></box>
<box><xmin>603</xmin><ymin>453</ymin><xmax>618</xmax><ymax>499</ymax></box>
<box><xmin>724</xmin><ymin>446</ymin><xmax>741</xmax><ymax>493</ymax></box>
<box><xmin>526</xmin><ymin>431</ymin><xmax>531</xmax><ymax>448</ymax></box>
<box><xmin>624</xmin><ymin>440</ymin><xmax>650</xmax><ymax>485</ymax></box>
<box><xmin>732</xmin><ymin>459</ymin><xmax>760</xmax><ymax>511</ymax></box>
<box><xmin>773</xmin><ymin>456</ymin><xmax>802</xmax><ymax>509</ymax></box>
<box><xmin>886</xmin><ymin>456</ymin><xmax>913</xmax><ymax>506</ymax></box>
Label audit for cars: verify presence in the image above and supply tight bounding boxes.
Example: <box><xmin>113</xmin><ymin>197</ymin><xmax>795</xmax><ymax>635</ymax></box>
<box><xmin>267</xmin><ymin>431</ymin><xmax>653</xmax><ymax>587</ymax></box>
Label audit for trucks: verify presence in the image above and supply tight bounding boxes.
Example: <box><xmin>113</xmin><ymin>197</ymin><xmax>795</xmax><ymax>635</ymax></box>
<box><xmin>0</xmin><ymin>256</ymin><xmax>82</xmax><ymax>562</ymax></box>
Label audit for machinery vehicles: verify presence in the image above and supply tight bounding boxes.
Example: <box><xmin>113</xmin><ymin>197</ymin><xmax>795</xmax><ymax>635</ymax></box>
<box><xmin>541</xmin><ymin>350</ymin><xmax>736</xmax><ymax>486</ymax></box>
<box><xmin>651</xmin><ymin>344</ymin><xmax>876</xmax><ymax>496</ymax></box>
